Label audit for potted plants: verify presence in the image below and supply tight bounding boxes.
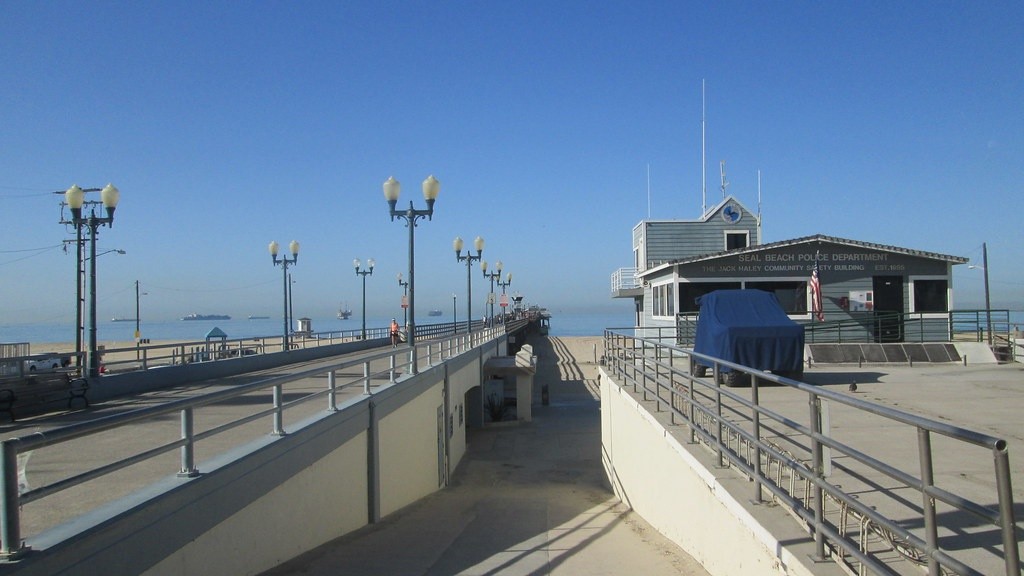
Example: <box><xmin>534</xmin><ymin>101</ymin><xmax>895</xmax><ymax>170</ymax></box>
<box><xmin>484</xmin><ymin>391</ymin><xmax>508</xmax><ymax>422</ymax></box>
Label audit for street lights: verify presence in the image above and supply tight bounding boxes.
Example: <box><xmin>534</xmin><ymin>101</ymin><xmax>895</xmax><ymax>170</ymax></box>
<box><xmin>353</xmin><ymin>257</ymin><xmax>376</xmax><ymax>340</ymax></box>
<box><xmin>967</xmin><ymin>264</ymin><xmax>993</xmax><ymax>351</ymax></box>
<box><xmin>382</xmin><ymin>174</ymin><xmax>440</xmax><ymax>375</ymax></box>
<box><xmin>289</xmin><ymin>279</ymin><xmax>297</xmax><ymax>350</ymax></box>
<box><xmin>64</xmin><ymin>181</ymin><xmax>120</xmax><ymax>379</ymax></box>
<box><xmin>136</xmin><ymin>292</ymin><xmax>149</xmax><ymax>361</ymax></box>
<box><xmin>453</xmin><ymin>235</ymin><xmax>484</xmax><ymax>334</ymax></box>
<box><xmin>269</xmin><ymin>239</ymin><xmax>299</xmax><ymax>351</ymax></box>
<box><xmin>481</xmin><ymin>259</ymin><xmax>504</xmax><ymax>328</ymax></box>
<box><xmin>397</xmin><ymin>272</ymin><xmax>409</xmax><ymax>330</ymax></box>
<box><xmin>451</xmin><ymin>292</ymin><xmax>459</xmax><ymax>333</ymax></box>
<box><xmin>495</xmin><ymin>272</ymin><xmax>513</xmax><ymax>325</ymax></box>
<box><xmin>75</xmin><ymin>247</ymin><xmax>128</xmax><ymax>372</ymax></box>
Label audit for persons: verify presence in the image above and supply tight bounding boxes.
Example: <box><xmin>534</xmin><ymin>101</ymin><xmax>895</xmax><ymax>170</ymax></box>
<box><xmin>390</xmin><ymin>318</ymin><xmax>398</xmax><ymax>348</ymax></box>
<box><xmin>406</xmin><ymin>320</ymin><xmax>416</xmax><ymax>333</ymax></box>
<box><xmin>488</xmin><ymin>317</ymin><xmax>491</xmax><ymax>328</ymax></box>
<box><xmin>498</xmin><ymin>314</ymin><xmax>509</xmax><ymax>324</ymax></box>
<box><xmin>99</xmin><ymin>365</ymin><xmax>106</xmax><ymax>373</ymax></box>
<box><xmin>482</xmin><ymin>315</ymin><xmax>486</xmax><ymax>328</ymax></box>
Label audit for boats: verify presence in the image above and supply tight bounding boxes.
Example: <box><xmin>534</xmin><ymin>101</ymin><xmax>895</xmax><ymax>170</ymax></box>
<box><xmin>111</xmin><ymin>316</ymin><xmax>142</xmax><ymax>322</ymax></box>
<box><xmin>336</xmin><ymin>308</ymin><xmax>353</xmax><ymax>320</ymax></box>
<box><xmin>180</xmin><ymin>311</ymin><xmax>232</xmax><ymax>321</ymax></box>
<box><xmin>248</xmin><ymin>313</ymin><xmax>271</xmax><ymax>319</ymax></box>
<box><xmin>427</xmin><ymin>309</ymin><xmax>443</xmax><ymax>316</ymax></box>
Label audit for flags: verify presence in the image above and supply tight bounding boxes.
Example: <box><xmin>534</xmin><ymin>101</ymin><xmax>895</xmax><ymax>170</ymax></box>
<box><xmin>810</xmin><ymin>256</ymin><xmax>824</xmax><ymax>322</ymax></box>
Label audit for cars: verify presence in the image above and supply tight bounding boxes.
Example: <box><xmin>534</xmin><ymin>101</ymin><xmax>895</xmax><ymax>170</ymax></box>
<box><xmin>11</xmin><ymin>352</ymin><xmax>62</xmax><ymax>375</ymax></box>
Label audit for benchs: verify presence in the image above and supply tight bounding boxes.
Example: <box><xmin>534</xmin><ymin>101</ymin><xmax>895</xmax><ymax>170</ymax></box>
<box><xmin>0</xmin><ymin>371</ymin><xmax>92</xmax><ymax>424</ymax></box>
